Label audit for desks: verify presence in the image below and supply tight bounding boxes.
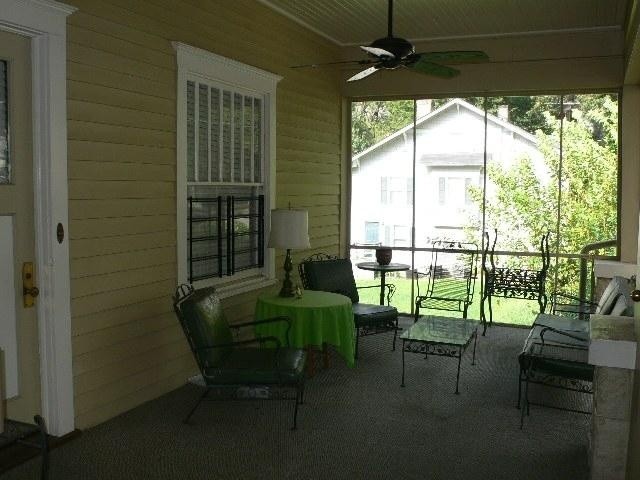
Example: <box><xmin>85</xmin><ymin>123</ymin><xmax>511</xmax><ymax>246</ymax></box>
<box><xmin>399</xmin><ymin>315</ymin><xmax>481</xmax><ymax>395</ymax></box>
<box><xmin>254</xmin><ymin>289</ymin><xmax>358</xmax><ymax>378</ymax></box>
<box><xmin>355</xmin><ymin>261</ymin><xmax>410</xmax><ymax>306</ymax></box>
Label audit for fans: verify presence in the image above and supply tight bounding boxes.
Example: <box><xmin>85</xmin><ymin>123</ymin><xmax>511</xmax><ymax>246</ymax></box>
<box><xmin>288</xmin><ymin>0</ymin><xmax>491</xmax><ymax>84</ymax></box>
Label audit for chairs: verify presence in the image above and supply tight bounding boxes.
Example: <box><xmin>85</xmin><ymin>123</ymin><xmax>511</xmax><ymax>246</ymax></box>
<box><xmin>413</xmin><ymin>241</ymin><xmax>479</xmax><ymax>324</ymax></box>
<box><xmin>298</xmin><ymin>256</ymin><xmax>399</xmax><ymax>357</ymax></box>
<box><xmin>174</xmin><ymin>284</ymin><xmax>309</xmax><ymax>430</ymax></box>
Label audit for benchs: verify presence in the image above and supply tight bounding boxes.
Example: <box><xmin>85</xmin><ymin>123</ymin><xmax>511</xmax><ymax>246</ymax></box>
<box><xmin>517</xmin><ymin>275</ymin><xmax>635</xmax><ymax>429</ymax></box>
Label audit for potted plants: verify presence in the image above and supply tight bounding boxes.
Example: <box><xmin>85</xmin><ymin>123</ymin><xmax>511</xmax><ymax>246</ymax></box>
<box><xmin>375</xmin><ymin>242</ymin><xmax>393</xmax><ymax>265</ymax></box>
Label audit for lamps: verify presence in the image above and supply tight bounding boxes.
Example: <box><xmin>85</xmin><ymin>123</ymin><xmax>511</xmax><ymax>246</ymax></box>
<box><xmin>266</xmin><ymin>200</ymin><xmax>312</xmax><ymax>297</ymax></box>
